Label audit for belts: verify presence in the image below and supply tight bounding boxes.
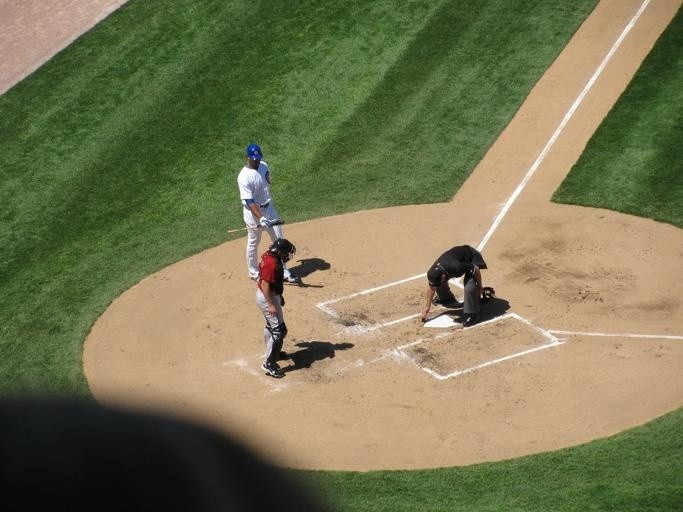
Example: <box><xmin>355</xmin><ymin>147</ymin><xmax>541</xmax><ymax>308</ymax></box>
<box><xmin>260</xmin><ymin>202</ymin><xmax>269</xmax><ymax>208</ymax></box>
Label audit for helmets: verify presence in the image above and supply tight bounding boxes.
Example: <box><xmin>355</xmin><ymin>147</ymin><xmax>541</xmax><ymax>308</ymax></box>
<box><xmin>269</xmin><ymin>238</ymin><xmax>296</xmax><ymax>263</ymax></box>
<box><xmin>247</xmin><ymin>145</ymin><xmax>263</xmax><ymax>161</ymax></box>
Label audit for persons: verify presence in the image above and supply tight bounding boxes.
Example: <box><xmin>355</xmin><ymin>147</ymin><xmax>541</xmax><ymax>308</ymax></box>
<box><xmin>421</xmin><ymin>245</ymin><xmax>488</xmax><ymax>327</ymax></box>
<box><xmin>255</xmin><ymin>238</ymin><xmax>297</xmax><ymax>377</ymax></box>
<box><xmin>237</xmin><ymin>144</ymin><xmax>301</xmax><ymax>283</ymax></box>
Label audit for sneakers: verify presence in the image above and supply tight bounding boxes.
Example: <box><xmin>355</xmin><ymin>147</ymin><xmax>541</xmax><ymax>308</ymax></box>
<box><xmin>432</xmin><ymin>296</ymin><xmax>454</xmax><ymax>304</ymax></box>
<box><xmin>251</xmin><ymin>275</ymin><xmax>260</xmax><ymax>282</ymax></box>
<box><xmin>285</xmin><ymin>274</ymin><xmax>298</xmax><ymax>283</ymax></box>
<box><xmin>463</xmin><ymin>315</ymin><xmax>475</xmax><ymax>326</ymax></box>
<box><xmin>275</xmin><ymin>351</ymin><xmax>285</xmax><ymax>360</ymax></box>
<box><xmin>262</xmin><ymin>360</ymin><xmax>284</xmax><ymax>378</ymax></box>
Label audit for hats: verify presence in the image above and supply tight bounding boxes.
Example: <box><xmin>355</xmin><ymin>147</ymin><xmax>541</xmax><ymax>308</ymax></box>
<box><xmin>428</xmin><ymin>269</ymin><xmax>443</xmax><ymax>286</ymax></box>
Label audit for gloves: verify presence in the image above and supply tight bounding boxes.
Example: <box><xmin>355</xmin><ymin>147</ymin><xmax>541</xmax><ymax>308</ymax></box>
<box><xmin>259</xmin><ymin>216</ymin><xmax>273</xmax><ymax>229</ymax></box>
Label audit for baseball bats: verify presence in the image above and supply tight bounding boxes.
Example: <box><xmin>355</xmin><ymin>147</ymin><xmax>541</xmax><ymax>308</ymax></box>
<box><xmin>227</xmin><ymin>219</ymin><xmax>284</xmax><ymax>233</ymax></box>
